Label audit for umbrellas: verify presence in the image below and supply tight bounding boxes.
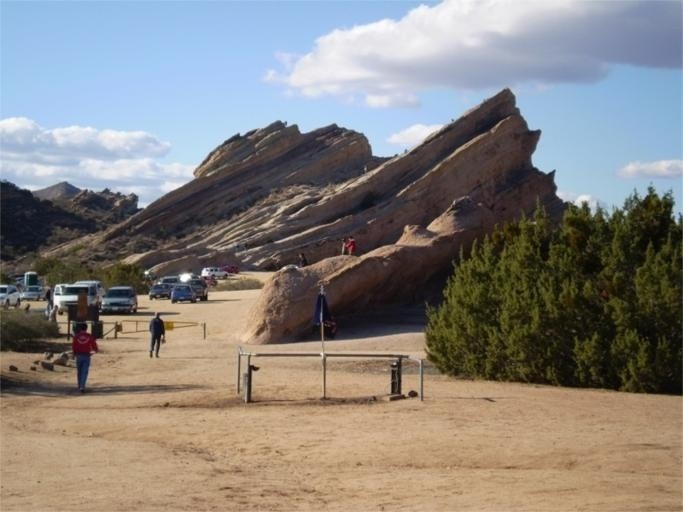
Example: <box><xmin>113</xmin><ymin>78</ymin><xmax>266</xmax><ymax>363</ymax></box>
<box><xmin>313</xmin><ymin>285</ymin><xmax>329</xmax><ymax>352</ymax></box>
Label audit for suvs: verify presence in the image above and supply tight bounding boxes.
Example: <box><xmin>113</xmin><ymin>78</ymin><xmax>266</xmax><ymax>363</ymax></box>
<box><xmin>0</xmin><ymin>284</ymin><xmax>22</xmax><ymax>309</ymax></box>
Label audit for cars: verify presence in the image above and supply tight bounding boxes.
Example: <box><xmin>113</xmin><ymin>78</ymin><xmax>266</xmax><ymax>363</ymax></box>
<box><xmin>201</xmin><ymin>267</ymin><xmax>228</xmax><ymax>280</ymax></box>
<box><xmin>22</xmin><ymin>285</ymin><xmax>45</xmax><ymax>301</ymax></box>
<box><xmin>51</xmin><ymin>279</ymin><xmax>138</xmax><ymax>315</ymax></box>
<box><xmin>149</xmin><ymin>275</ymin><xmax>208</xmax><ymax>303</ymax></box>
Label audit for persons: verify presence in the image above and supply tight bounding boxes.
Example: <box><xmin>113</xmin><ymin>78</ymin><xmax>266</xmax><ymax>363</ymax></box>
<box><xmin>148</xmin><ymin>312</ymin><xmax>166</xmax><ymax>357</ymax></box>
<box><xmin>48</xmin><ymin>305</ymin><xmax>58</xmax><ymax>321</ymax></box>
<box><xmin>44</xmin><ymin>285</ymin><xmax>54</xmax><ymax>315</ymax></box>
<box><xmin>72</xmin><ymin>323</ymin><xmax>98</xmax><ymax>392</ymax></box>
<box><xmin>341</xmin><ymin>236</ymin><xmax>355</xmax><ymax>256</ymax></box>
<box><xmin>299</xmin><ymin>252</ymin><xmax>307</xmax><ymax>267</ymax></box>
<box><xmin>325</xmin><ymin>315</ymin><xmax>337</xmax><ymax>339</ymax></box>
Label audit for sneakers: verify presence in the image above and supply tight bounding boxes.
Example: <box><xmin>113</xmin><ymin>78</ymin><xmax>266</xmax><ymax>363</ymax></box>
<box><xmin>79</xmin><ymin>386</ymin><xmax>85</xmax><ymax>392</ymax></box>
